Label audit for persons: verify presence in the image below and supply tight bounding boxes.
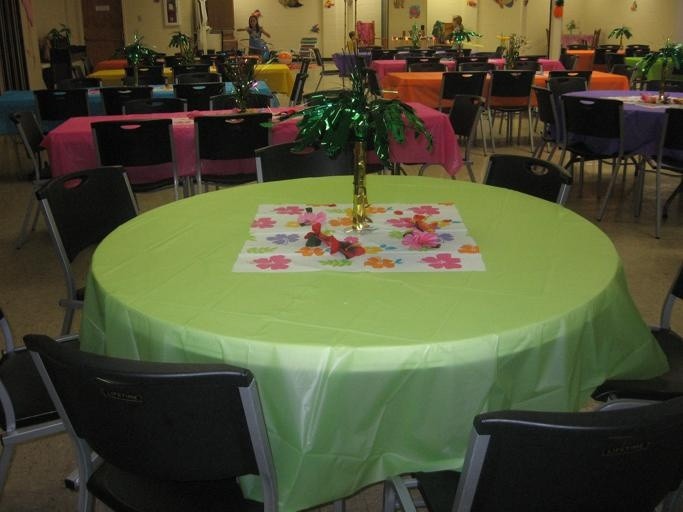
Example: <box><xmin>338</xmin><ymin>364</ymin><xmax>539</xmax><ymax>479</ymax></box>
<box><xmin>452</xmin><ymin>15</ymin><xmax>464</xmax><ymax>32</ymax></box>
<box><xmin>344</xmin><ymin>30</ymin><xmax>363</xmax><ymax>54</ymax></box>
<box><xmin>236</xmin><ymin>15</ymin><xmax>271</xmax><ymax>64</ymax></box>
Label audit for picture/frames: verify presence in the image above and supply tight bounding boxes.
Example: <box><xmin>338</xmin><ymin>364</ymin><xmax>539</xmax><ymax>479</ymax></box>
<box><xmin>162</xmin><ymin>0</ymin><xmax>182</xmax><ymax>28</ymax></box>
